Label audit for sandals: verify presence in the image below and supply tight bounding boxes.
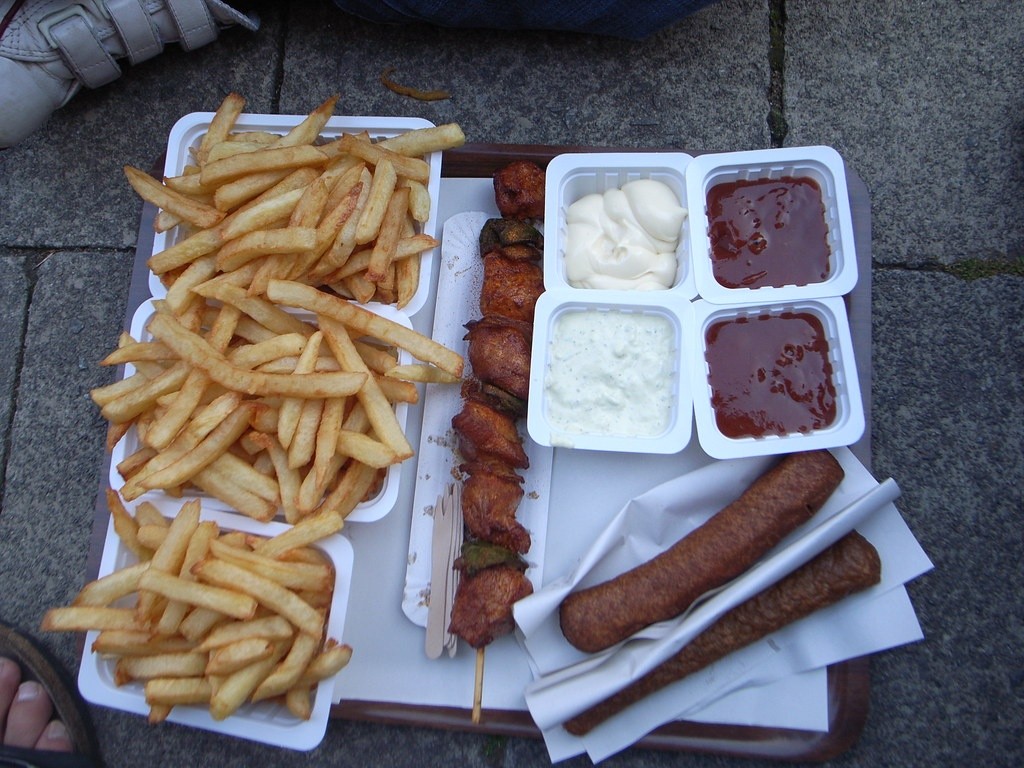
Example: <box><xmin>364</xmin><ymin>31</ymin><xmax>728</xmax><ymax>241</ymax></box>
<box><xmin>0</xmin><ymin>619</ymin><xmax>106</xmax><ymax>768</ymax></box>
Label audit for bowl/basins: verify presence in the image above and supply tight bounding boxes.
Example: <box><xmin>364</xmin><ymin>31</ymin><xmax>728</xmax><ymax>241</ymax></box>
<box><xmin>526</xmin><ymin>145</ymin><xmax>866</xmax><ymax>459</ymax></box>
<box><xmin>77</xmin><ymin>502</ymin><xmax>354</xmax><ymax>752</ymax></box>
<box><xmin>107</xmin><ymin>297</ymin><xmax>416</xmax><ymax>522</ymax></box>
<box><xmin>148</xmin><ymin>113</ymin><xmax>441</xmax><ymax>318</ymax></box>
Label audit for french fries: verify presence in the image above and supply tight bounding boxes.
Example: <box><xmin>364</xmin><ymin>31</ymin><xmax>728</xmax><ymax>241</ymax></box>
<box><xmin>38</xmin><ymin>95</ymin><xmax>465</xmax><ymax>724</ymax></box>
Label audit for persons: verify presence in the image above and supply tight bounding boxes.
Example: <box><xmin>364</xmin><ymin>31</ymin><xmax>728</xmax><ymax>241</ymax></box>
<box><xmin>0</xmin><ymin>620</ymin><xmax>102</xmax><ymax>768</ymax></box>
<box><xmin>0</xmin><ymin>0</ymin><xmax>721</xmax><ymax>149</ymax></box>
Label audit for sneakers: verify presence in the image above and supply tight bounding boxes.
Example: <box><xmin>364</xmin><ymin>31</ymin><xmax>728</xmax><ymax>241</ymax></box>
<box><xmin>0</xmin><ymin>0</ymin><xmax>265</xmax><ymax>148</ymax></box>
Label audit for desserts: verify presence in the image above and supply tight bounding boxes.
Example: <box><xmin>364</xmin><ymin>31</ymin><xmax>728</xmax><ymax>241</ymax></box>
<box><xmin>563</xmin><ymin>179</ymin><xmax>690</xmax><ymax>292</ymax></box>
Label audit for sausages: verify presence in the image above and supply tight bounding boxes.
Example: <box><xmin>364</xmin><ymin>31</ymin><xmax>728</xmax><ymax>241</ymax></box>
<box><xmin>559</xmin><ymin>444</ymin><xmax>844</xmax><ymax>652</ymax></box>
<box><xmin>563</xmin><ymin>530</ymin><xmax>884</xmax><ymax>737</ymax></box>
